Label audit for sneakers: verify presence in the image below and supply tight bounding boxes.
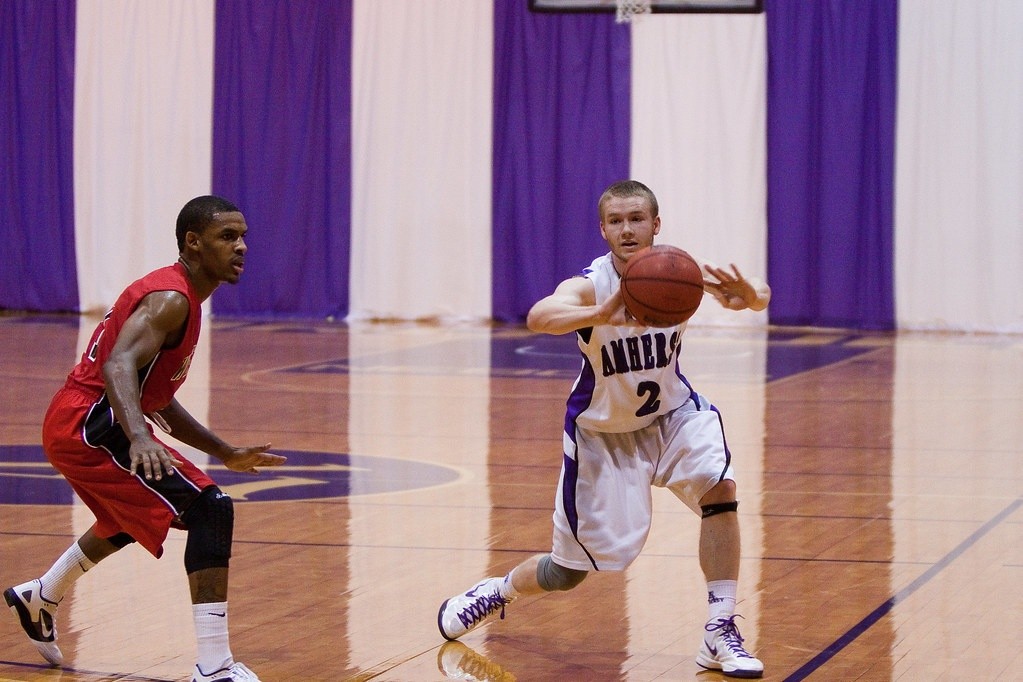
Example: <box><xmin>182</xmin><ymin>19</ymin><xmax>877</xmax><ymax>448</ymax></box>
<box><xmin>438</xmin><ymin>577</ymin><xmax>517</xmax><ymax>641</ymax></box>
<box><xmin>191</xmin><ymin>661</ymin><xmax>261</xmax><ymax>682</ymax></box>
<box><xmin>695</xmin><ymin>614</ymin><xmax>765</xmax><ymax>677</ymax></box>
<box><xmin>437</xmin><ymin>641</ymin><xmax>516</xmax><ymax>682</ymax></box>
<box><xmin>2</xmin><ymin>578</ymin><xmax>63</xmax><ymax>666</ymax></box>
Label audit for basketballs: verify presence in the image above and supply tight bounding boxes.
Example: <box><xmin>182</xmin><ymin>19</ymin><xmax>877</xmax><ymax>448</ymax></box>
<box><xmin>620</xmin><ymin>245</ymin><xmax>704</xmax><ymax>329</ymax></box>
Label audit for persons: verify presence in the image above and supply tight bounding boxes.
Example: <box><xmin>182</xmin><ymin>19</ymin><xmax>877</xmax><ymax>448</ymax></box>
<box><xmin>4</xmin><ymin>197</ymin><xmax>287</xmax><ymax>682</ymax></box>
<box><xmin>438</xmin><ymin>181</ymin><xmax>772</xmax><ymax>677</ymax></box>
